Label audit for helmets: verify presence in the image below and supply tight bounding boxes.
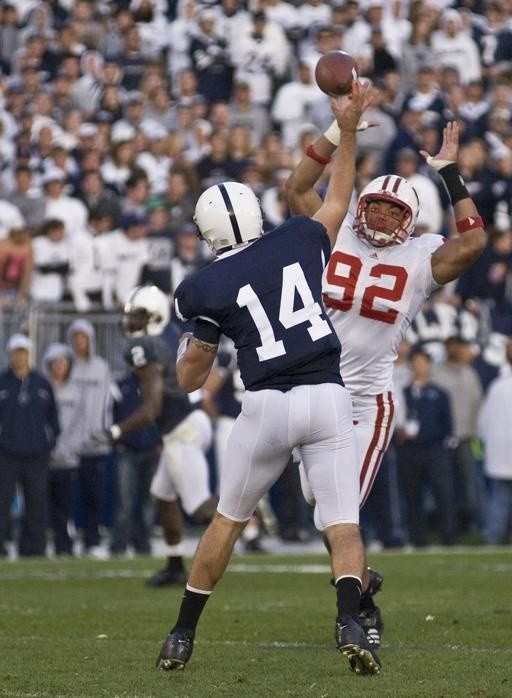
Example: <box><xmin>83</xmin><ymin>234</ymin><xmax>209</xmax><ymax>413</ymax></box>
<box><xmin>121</xmin><ymin>285</ymin><xmax>171</xmax><ymax>337</ymax></box>
<box><xmin>192</xmin><ymin>181</ymin><xmax>265</xmax><ymax>257</ymax></box>
<box><xmin>352</xmin><ymin>174</ymin><xmax>422</xmax><ymax>248</ymax></box>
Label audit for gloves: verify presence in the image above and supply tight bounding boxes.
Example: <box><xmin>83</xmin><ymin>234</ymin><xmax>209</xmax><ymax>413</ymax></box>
<box><xmin>89</xmin><ymin>428</ymin><xmax>113</xmax><ymax>448</ymax></box>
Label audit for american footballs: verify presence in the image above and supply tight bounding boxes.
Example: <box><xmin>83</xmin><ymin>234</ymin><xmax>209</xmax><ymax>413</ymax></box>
<box><xmin>316</xmin><ymin>53</ymin><xmax>358</xmax><ymax>96</ymax></box>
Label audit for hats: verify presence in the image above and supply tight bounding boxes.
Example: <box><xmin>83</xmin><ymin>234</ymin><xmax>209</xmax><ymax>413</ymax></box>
<box><xmin>121</xmin><ymin>337</ymin><xmax>162</xmax><ymax>371</ymax></box>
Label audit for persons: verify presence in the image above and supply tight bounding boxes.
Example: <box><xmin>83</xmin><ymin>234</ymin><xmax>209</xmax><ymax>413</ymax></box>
<box><xmin>283</xmin><ymin>78</ymin><xmax>490</xmax><ymax>654</ymax></box>
<box><xmin>1</xmin><ymin>0</ymin><xmax>512</xmax><ymax>591</ymax></box>
<box><xmin>153</xmin><ymin>77</ymin><xmax>389</xmax><ymax>677</ymax></box>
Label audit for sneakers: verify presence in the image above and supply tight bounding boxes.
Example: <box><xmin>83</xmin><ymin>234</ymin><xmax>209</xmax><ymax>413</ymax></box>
<box><xmin>145</xmin><ymin>572</ymin><xmax>187</xmax><ymax>590</ymax></box>
<box><xmin>334</xmin><ymin>617</ymin><xmax>382</xmax><ymax>677</ymax></box>
<box><xmin>359</xmin><ymin>568</ymin><xmax>384</xmax><ymax>608</ymax></box>
<box><xmin>360</xmin><ymin>606</ymin><xmax>384</xmax><ymax>650</ymax></box>
<box><xmin>155</xmin><ymin>632</ymin><xmax>193</xmax><ymax>670</ymax></box>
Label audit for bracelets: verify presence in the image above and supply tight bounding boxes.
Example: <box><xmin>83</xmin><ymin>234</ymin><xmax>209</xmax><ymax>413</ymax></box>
<box><xmin>454</xmin><ymin>216</ymin><xmax>485</xmax><ymax>233</ymax></box>
<box><xmin>438</xmin><ymin>162</ymin><xmax>472</xmax><ymax>205</ymax></box>
<box><xmin>306</xmin><ymin>142</ymin><xmax>333</xmax><ymax>167</ymax></box>
